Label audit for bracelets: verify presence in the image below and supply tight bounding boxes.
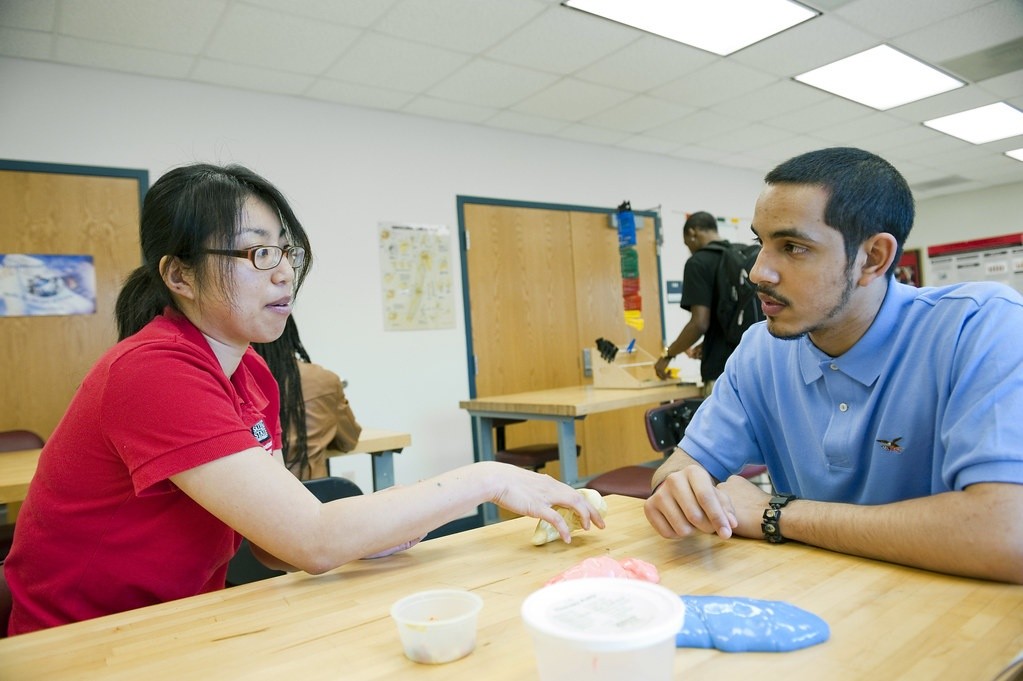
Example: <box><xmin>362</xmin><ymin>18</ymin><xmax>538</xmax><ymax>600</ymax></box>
<box><xmin>661</xmin><ymin>347</ymin><xmax>675</xmax><ymax>360</ymax></box>
<box><xmin>760</xmin><ymin>493</ymin><xmax>799</xmax><ymax>544</ymax></box>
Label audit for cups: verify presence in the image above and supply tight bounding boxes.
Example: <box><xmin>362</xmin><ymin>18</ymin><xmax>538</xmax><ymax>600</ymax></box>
<box><xmin>522</xmin><ymin>576</ymin><xmax>686</xmax><ymax>680</ymax></box>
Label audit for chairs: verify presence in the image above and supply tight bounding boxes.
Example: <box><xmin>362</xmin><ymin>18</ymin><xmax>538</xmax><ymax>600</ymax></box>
<box><xmin>587</xmin><ymin>397</ymin><xmax>707</xmax><ymax>499</ymax></box>
<box><xmin>488</xmin><ymin>414</ymin><xmax>581</xmax><ymax>472</ymax></box>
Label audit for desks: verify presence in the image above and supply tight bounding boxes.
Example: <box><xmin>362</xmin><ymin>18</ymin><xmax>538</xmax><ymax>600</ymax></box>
<box><xmin>1</xmin><ymin>431</ymin><xmax>410</xmax><ymax>527</ymax></box>
<box><xmin>0</xmin><ymin>493</ymin><xmax>1023</xmax><ymax>681</ymax></box>
<box><xmin>458</xmin><ymin>386</ymin><xmax>704</xmax><ymax>523</ymax></box>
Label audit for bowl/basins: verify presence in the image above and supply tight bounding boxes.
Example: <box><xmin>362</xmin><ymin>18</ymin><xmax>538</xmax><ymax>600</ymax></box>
<box><xmin>389</xmin><ymin>588</ymin><xmax>484</xmax><ymax>664</ymax></box>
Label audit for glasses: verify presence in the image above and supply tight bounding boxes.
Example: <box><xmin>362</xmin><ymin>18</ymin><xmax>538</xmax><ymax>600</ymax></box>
<box><xmin>196</xmin><ymin>245</ymin><xmax>307</xmax><ymax>270</ymax></box>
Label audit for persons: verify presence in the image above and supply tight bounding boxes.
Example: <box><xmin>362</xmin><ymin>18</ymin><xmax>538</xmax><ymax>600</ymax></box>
<box><xmin>644</xmin><ymin>147</ymin><xmax>1022</xmax><ymax>588</ymax></box>
<box><xmin>654</xmin><ymin>210</ymin><xmax>740</xmax><ymax>397</ymax></box>
<box><xmin>250</xmin><ymin>313</ymin><xmax>362</xmax><ymax>481</ymax></box>
<box><xmin>1</xmin><ymin>163</ymin><xmax>606</xmax><ymax>638</ymax></box>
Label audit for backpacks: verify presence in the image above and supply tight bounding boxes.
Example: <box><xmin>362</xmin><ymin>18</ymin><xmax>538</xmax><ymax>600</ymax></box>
<box><xmin>696</xmin><ymin>243</ymin><xmax>768</xmax><ymax>347</ymax></box>
